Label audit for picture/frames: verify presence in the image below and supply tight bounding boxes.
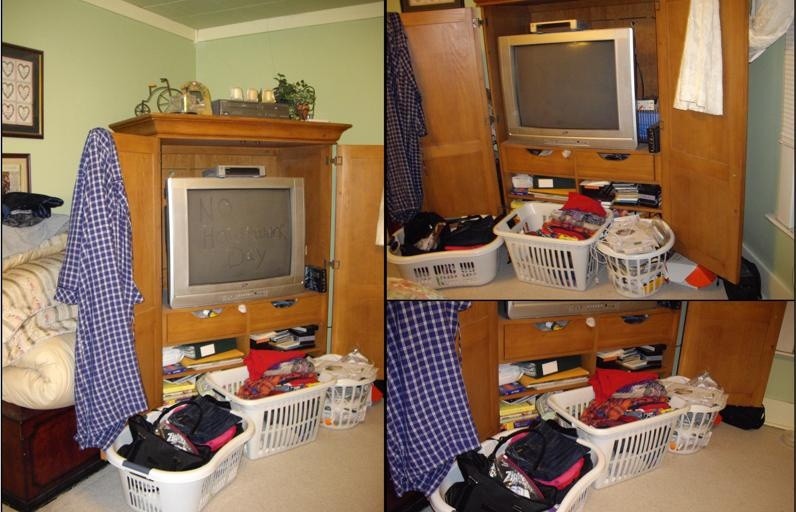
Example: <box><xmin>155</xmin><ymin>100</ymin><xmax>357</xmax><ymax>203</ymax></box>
<box><xmin>2</xmin><ymin>41</ymin><xmax>48</xmax><ymax>202</ymax></box>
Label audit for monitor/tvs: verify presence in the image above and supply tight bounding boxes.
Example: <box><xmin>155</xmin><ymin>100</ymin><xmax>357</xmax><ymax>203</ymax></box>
<box><xmin>497</xmin><ymin>27</ymin><xmax>639</xmax><ymax>150</ymax></box>
<box><xmin>165</xmin><ymin>177</ymin><xmax>306</xmax><ymax>308</ymax></box>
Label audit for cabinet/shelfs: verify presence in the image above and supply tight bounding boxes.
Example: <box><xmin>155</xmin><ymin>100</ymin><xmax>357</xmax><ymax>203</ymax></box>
<box><xmin>445</xmin><ymin>299</ymin><xmax>788</xmax><ymax>459</ymax></box>
<box><xmin>82</xmin><ymin>114</ymin><xmax>383</xmax><ymax>450</ymax></box>
<box><xmin>385</xmin><ymin>0</ymin><xmax>750</xmax><ymax>286</ymax></box>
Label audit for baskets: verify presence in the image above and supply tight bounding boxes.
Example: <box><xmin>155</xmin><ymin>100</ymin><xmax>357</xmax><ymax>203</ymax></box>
<box><xmin>204</xmin><ymin>367</ymin><xmax>336</xmax><ymax>461</ymax></box>
<box><xmin>597</xmin><ymin>216</ymin><xmax>675</xmax><ymax>298</ymax></box>
<box><xmin>671</xmin><ymin>405</ymin><xmax>726</xmax><ymax>455</ymax></box>
<box><xmin>430</xmin><ymin>427</ymin><xmax>605</xmax><ymax>512</ymax></box>
<box><xmin>548</xmin><ymin>379</ymin><xmax>690</xmax><ymax>491</ymax></box>
<box><xmin>323</xmin><ymin>376</ymin><xmax>378</xmax><ymax>429</ymax></box>
<box><xmin>386</xmin><ymin>214</ymin><xmax>503</xmax><ymax>289</ymax></box>
<box><xmin>105</xmin><ymin>401</ymin><xmax>255</xmax><ymax>512</ymax></box>
<box><xmin>494</xmin><ymin>201</ymin><xmax>609</xmax><ymax>291</ymax></box>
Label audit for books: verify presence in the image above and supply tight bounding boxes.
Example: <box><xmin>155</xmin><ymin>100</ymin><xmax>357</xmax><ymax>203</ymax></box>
<box><xmin>164</xmin><ymin>340</ymin><xmax>244</xmax><ymax>403</ymax></box>
<box><xmin>249</xmin><ymin>325</ymin><xmax>320</xmax><ymax>353</ymax></box>
<box><xmin>527</xmin><ymin>177</ymin><xmax>663</xmax><ymax>209</ymax></box>
<box><xmin>498</xmin><ymin>345</ymin><xmax>666</xmax><ymax>430</ymax></box>
